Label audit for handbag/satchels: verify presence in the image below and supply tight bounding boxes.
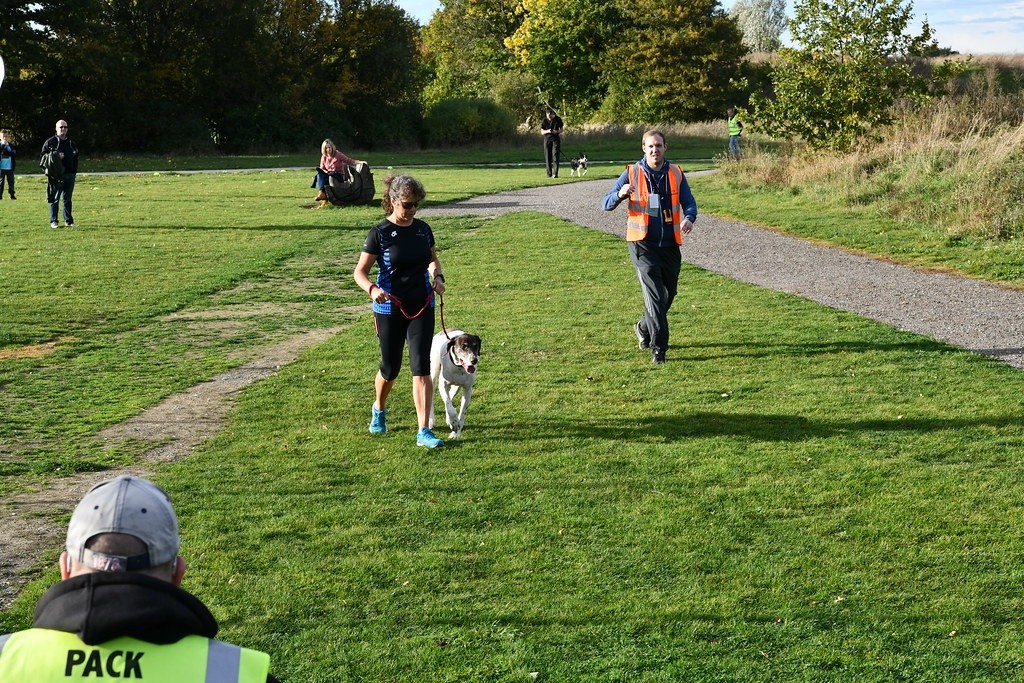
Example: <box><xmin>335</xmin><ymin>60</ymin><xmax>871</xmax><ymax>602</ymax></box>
<box><xmin>0</xmin><ymin>158</ymin><xmax>12</xmax><ymax>170</ymax></box>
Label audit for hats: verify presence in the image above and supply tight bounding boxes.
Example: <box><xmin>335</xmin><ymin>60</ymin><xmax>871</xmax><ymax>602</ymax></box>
<box><xmin>66</xmin><ymin>475</ymin><xmax>180</xmax><ymax>573</ymax></box>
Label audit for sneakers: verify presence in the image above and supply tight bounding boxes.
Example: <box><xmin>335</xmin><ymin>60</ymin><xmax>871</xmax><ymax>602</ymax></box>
<box><xmin>369</xmin><ymin>401</ymin><xmax>386</xmax><ymax>434</ymax></box>
<box><xmin>417</xmin><ymin>427</ymin><xmax>444</xmax><ymax>449</ymax></box>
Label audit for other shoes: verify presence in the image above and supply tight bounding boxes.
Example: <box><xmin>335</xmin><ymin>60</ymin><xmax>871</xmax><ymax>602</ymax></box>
<box><xmin>315</xmin><ymin>195</ymin><xmax>327</xmax><ymax>201</ymax></box>
<box><xmin>65</xmin><ymin>223</ymin><xmax>79</xmax><ymax>227</ymax></box>
<box><xmin>51</xmin><ymin>221</ymin><xmax>59</xmax><ymax>229</ymax></box>
<box><xmin>0</xmin><ymin>195</ymin><xmax>2</xmax><ymax>199</ymax></box>
<box><xmin>634</xmin><ymin>323</ymin><xmax>649</xmax><ymax>350</ymax></box>
<box><xmin>11</xmin><ymin>194</ymin><xmax>16</xmax><ymax>199</ymax></box>
<box><xmin>652</xmin><ymin>355</ymin><xmax>665</xmax><ymax>364</ymax></box>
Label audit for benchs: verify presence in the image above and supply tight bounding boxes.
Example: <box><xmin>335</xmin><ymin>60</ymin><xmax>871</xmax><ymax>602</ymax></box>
<box><xmin>323</xmin><ymin>159</ymin><xmax>376</xmax><ymax>206</ymax></box>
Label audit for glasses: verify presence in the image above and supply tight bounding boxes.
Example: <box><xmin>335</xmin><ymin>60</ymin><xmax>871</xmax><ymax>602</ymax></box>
<box><xmin>397</xmin><ymin>197</ymin><xmax>419</xmax><ymax>209</ymax></box>
<box><xmin>57</xmin><ymin>126</ymin><xmax>68</xmax><ymax>128</ymax></box>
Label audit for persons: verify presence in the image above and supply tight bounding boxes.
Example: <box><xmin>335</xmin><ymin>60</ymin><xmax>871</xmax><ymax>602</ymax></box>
<box><xmin>0</xmin><ymin>130</ymin><xmax>17</xmax><ymax>199</ymax></box>
<box><xmin>602</xmin><ymin>131</ymin><xmax>697</xmax><ymax>363</ymax></box>
<box><xmin>728</xmin><ymin>108</ymin><xmax>744</xmax><ymax>159</ymax></box>
<box><xmin>315</xmin><ymin>140</ymin><xmax>367</xmax><ymax>208</ymax></box>
<box><xmin>41</xmin><ymin>120</ymin><xmax>79</xmax><ymax>228</ymax></box>
<box><xmin>541</xmin><ymin>110</ymin><xmax>563</xmax><ymax>178</ymax></box>
<box><xmin>0</xmin><ymin>476</ymin><xmax>279</xmax><ymax>683</ymax></box>
<box><xmin>354</xmin><ymin>175</ymin><xmax>445</xmax><ymax>447</ymax></box>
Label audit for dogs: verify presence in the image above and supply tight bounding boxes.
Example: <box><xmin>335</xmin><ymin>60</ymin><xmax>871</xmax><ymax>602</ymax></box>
<box><xmin>571</xmin><ymin>152</ymin><xmax>589</xmax><ymax>177</ymax></box>
<box><xmin>430</xmin><ymin>326</ymin><xmax>482</xmax><ymax>440</ymax></box>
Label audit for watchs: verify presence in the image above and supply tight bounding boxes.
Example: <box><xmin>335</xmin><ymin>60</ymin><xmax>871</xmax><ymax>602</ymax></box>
<box><xmin>434</xmin><ymin>274</ymin><xmax>445</xmax><ymax>283</ymax></box>
